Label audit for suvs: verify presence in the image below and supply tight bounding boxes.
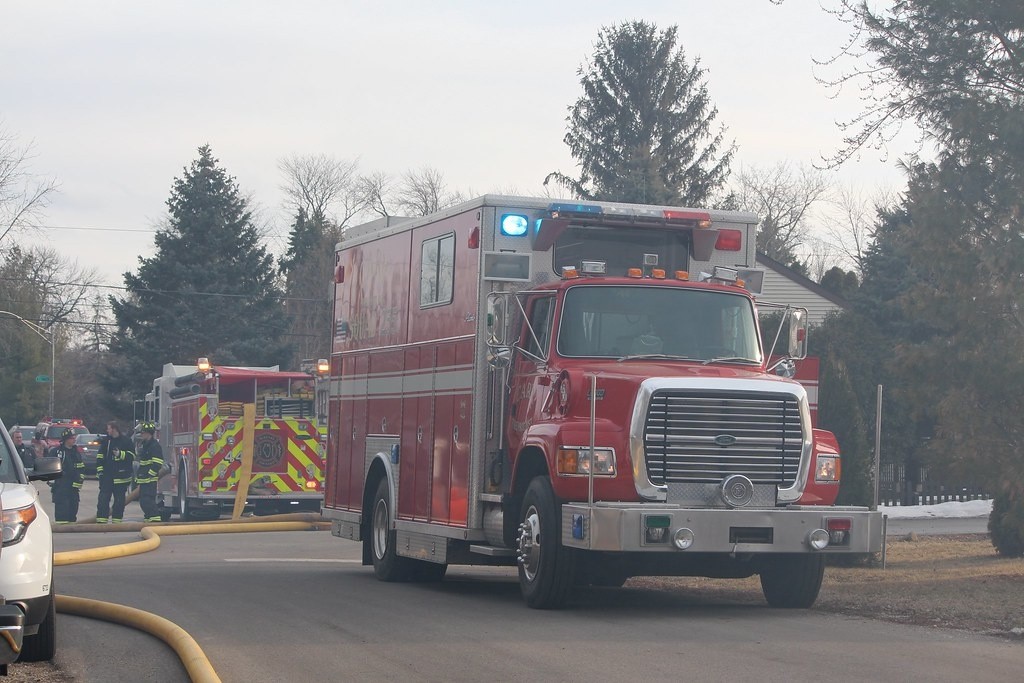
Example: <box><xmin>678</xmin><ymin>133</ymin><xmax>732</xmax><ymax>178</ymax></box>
<box><xmin>31</xmin><ymin>416</ymin><xmax>91</xmax><ymax>457</ymax></box>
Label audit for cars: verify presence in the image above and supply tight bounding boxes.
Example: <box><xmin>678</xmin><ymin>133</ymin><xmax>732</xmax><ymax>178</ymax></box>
<box><xmin>7</xmin><ymin>422</ymin><xmax>38</xmax><ymax>448</ymax></box>
<box><xmin>72</xmin><ymin>434</ymin><xmax>108</xmax><ymax>475</ymax></box>
<box><xmin>0</xmin><ymin>421</ymin><xmax>63</xmax><ymax>661</ymax></box>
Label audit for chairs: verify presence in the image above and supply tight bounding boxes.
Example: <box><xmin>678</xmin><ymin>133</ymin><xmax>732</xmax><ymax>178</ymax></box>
<box><xmin>657</xmin><ymin>296</ymin><xmax>723</xmax><ymax>358</ymax></box>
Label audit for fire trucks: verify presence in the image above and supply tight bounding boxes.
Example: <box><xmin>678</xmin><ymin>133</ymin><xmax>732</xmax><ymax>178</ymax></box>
<box><xmin>320</xmin><ymin>193</ymin><xmax>885</xmax><ymax>612</ymax></box>
<box><xmin>131</xmin><ymin>356</ymin><xmax>330</xmax><ymax>523</ymax></box>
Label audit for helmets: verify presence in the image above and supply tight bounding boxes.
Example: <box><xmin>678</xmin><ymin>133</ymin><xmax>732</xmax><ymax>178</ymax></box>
<box><xmin>139</xmin><ymin>422</ymin><xmax>156</xmax><ymax>433</ymax></box>
<box><xmin>59</xmin><ymin>427</ymin><xmax>79</xmax><ymax>442</ymax></box>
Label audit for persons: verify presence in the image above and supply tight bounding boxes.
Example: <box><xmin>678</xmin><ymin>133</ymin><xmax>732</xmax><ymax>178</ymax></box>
<box><xmin>46</xmin><ymin>427</ymin><xmax>86</xmax><ymax>523</ymax></box>
<box><xmin>137</xmin><ymin>423</ymin><xmax>163</xmax><ymax>523</ymax></box>
<box><xmin>96</xmin><ymin>421</ymin><xmax>136</xmax><ymax>524</ymax></box>
<box><xmin>11</xmin><ymin>429</ymin><xmax>34</xmax><ymax>472</ymax></box>
